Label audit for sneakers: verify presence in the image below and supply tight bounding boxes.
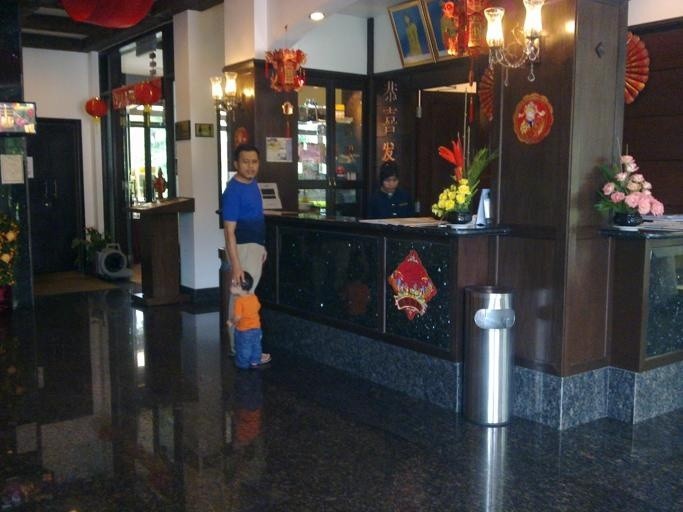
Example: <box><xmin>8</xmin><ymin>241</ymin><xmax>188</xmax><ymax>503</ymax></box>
<box><xmin>251</xmin><ymin>363</ymin><xmax>258</xmax><ymax>369</ymax></box>
<box><xmin>259</xmin><ymin>353</ymin><xmax>271</xmax><ymax>364</ymax></box>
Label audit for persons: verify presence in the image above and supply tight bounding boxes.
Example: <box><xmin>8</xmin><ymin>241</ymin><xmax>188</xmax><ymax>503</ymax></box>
<box><xmin>229</xmin><ymin>365</ymin><xmax>264</xmax><ymax>460</ymax></box>
<box><xmin>225</xmin><ymin>270</ymin><xmax>276</xmax><ymax>369</ymax></box>
<box><xmin>222</xmin><ymin>144</ymin><xmax>272</xmax><ymax>357</ymax></box>
<box><xmin>366</xmin><ymin>161</ymin><xmax>414</xmax><ymax>219</ymax></box>
<box><xmin>403</xmin><ymin>12</ymin><xmax>422</xmax><ymax>55</ymax></box>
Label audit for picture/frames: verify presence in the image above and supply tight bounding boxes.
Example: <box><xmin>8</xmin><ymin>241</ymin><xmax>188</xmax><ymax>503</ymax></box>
<box><xmin>386</xmin><ymin>0</ymin><xmax>437</xmax><ymax>68</ymax></box>
<box><xmin>421</xmin><ymin>0</ymin><xmax>469</xmax><ymax>63</ymax></box>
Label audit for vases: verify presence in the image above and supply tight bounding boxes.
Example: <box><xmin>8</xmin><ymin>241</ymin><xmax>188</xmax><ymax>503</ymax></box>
<box><xmin>0</xmin><ymin>284</ymin><xmax>13</xmax><ymax>316</ymax></box>
<box><xmin>446</xmin><ymin>212</ymin><xmax>473</xmax><ymax>224</ymax></box>
<box><xmin>612</xmin><ymin>207</ymin><xmax>644</xmax><ymax>226</ymax></box>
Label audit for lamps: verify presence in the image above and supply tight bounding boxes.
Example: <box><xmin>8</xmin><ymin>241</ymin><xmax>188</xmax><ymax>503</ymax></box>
<box><xmin>209</xmin><ymin>72</ymin><xmax>243</xmax><ymax>114</ymax></box>
<box><xmin>483</xmin><ymin>0</ymin><xmax>548</xmax><ymax>87</ymax></box>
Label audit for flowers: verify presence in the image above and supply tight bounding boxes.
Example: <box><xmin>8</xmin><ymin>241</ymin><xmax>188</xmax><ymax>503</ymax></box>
<box><xmin>0</xmin><ymin>210</ymin><xmax>22</xmax><ymax>287</ymax></box>
<box><xmin>591</xmin><ymin>138</ymin><xmax>666</xmax><ymax>217</ymax></box>
<box><xmin>431</xmin><ymin>132</ymin><xmax>499</xmax><ymax>219</ymax></box>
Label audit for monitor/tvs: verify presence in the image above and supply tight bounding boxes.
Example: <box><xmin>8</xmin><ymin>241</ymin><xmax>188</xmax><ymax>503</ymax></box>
<box><xmin>0</xmin><ymin>100</ymin><xmax>39</xmax><ymax>137</ymax></box>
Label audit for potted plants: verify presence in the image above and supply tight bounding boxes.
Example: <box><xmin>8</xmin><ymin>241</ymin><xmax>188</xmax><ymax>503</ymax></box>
<box><xmin>71</xmin><ymin>225</ymin><xmax>113</xmax><ymax>275</ymax></box>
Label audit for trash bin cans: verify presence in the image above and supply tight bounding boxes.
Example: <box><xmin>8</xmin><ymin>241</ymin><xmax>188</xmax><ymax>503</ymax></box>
<box><xmin>464</xmin><ymin>284</ymin><xmax>516</xmax><ymax>427</ymax></box>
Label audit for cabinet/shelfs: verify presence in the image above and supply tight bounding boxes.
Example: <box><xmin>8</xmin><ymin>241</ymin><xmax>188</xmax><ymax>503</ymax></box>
<box><xmin>222</xmin><ymin>58</ymin><xmax>369</xmax><ymax>216</ymax></box>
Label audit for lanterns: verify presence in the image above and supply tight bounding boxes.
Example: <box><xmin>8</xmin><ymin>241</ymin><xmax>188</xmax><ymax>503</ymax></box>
<box><xmin>135</xmin><ymin>82</ymin><xmax>161</xmax><ymax>132</ymax></box>
<box><xmin>86</xmin><ymin>97</ymin><xmax>108</xmax><ymax>124</ymax></box>
